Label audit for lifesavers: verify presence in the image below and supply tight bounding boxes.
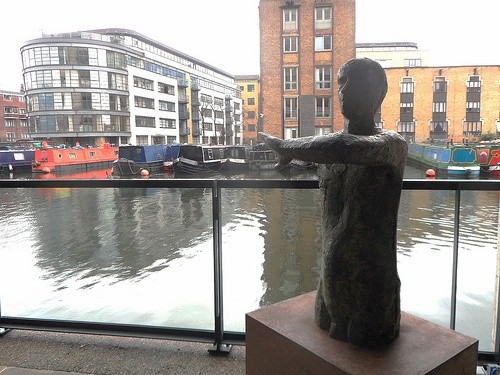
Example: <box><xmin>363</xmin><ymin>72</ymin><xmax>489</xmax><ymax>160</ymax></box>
<box><xmin>479</xmin><ymin>151</ymin><xmax>487</xmax><ymax>162</ymax></box>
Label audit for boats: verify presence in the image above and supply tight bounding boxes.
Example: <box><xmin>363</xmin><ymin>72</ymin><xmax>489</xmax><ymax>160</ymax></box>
<box><xmin>31</xmin><ymin>140</ymin><xmax>119</xmax><ymax>173</ymax></box>
<box><xmin>175</xmin><ymin>143</ymin><xmax>245</xmax><ymax>174</ymax></box>
<box><xmin>110</xmin><ymin>141</ymin><xmax>180</xmax><ymax>175</ymax></box>
<box><xmin>474</xmin><ymin>140</ymin><xmax>500</xmax><ymax>175</ymax></box>
<box><xmin>0</xmin><ymin>144</ymin><xmax>35</xmax><ymax>172</ymax></box>
<box><xmin>244</xmin><ymin>142</ymin><xmax>278</xmax><ymax>171</ymax></box>
<box><xmin>407</xmin><ymin>136</ymin><xmax>480</xmax><ymax>176</ymax></box>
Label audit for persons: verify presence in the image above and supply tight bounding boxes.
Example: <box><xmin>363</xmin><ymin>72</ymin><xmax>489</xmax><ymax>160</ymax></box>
<box><xmin>257</xmin><ymin>58</ymin><xmax>408</xmax><ymax>345</ymax></box>
